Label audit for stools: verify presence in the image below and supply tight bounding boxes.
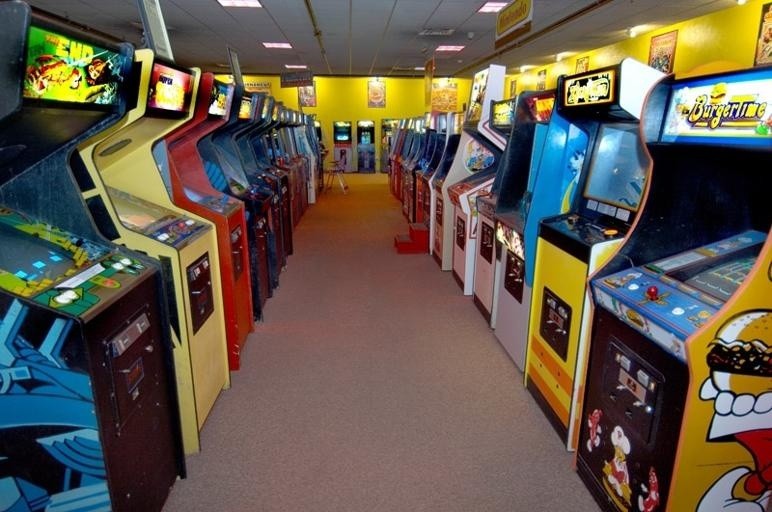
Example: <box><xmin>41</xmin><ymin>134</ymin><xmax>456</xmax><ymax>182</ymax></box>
<box><xmin>324</xmin><ymin>166</ymin><xmax>349</xmax><ymax>195</ymax></box>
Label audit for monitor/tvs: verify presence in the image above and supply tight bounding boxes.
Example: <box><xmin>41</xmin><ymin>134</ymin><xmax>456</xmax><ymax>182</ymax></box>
<box><xmin>184</xmin><ymin>186</ymin><xmax>202</xmax><ymax>202</ymax></box>
<box><xmin>335</xmin><ymin>127</ymin><xmax>349</xmax><ymax>141</ymax></box>
<box><xmin>108</xmin><ymin>193</ymin><xmax>165</xmax><ymax>234</ymax></box>
<box><xmin>680</xmin><ymin>245</ymin><xmax>759</xmax><ymax>300</ymax></box>
<box><xmin>360</xmin><ymin>131</ymin><xmax>371</xmax><ymax>144</ymax></box>
<box><xmin>0</xmin><ymin>222</ymin><xmax>75</xmax><ymax>283</ymax></box>
<box><xmin>583</xmin><ymin>123</ymin><xmax>651</xmax><ymax>211</ymax></box>
